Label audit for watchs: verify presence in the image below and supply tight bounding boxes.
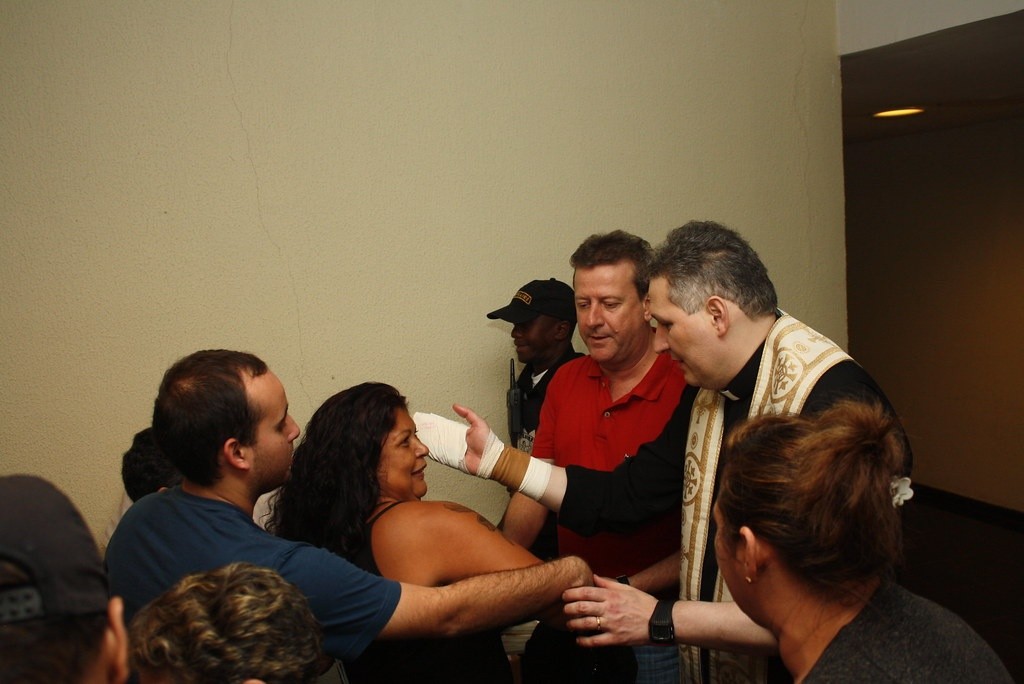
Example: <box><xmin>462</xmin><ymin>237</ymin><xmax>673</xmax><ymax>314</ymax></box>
<box><xmin>649</xmin><ymin>599</ymin><xmax>674</xmax><ymax>644</ymax></box>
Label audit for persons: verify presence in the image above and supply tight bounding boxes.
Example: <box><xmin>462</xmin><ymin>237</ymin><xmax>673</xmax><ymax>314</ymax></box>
<box><xmin>499</xmin><ymin>230</ymin><xmax>687</xmax><ymax>684</ymax></box>
<box><xmin>413</xmin><ymin>219</ymin><xmax>914</xmax><ymax>684</ymax></box>
<box><xmin>120</xmin><ymin>425</ymin><xmax>184</xmax><ymax>502</ymax></box>
<box><xmin>102</xmin><ymin>348</ymin><xmax>595</xmax><ymax>673</ymax></box>
<box><xmin>266</xmin><ymin>380</ymin><xmax>550</xmax><ymax>684</ymax></box>
<box><xmin>711</xmin><ymin>398</ymin><xmax>1013</xmax><ymax>684</ymax></box>
<box><xmin>487</xmin><ymin>278</ymin><xmax>589</xmax><ymax>561</ymax></box>
<box><xmin>0</xmin><ymin>473</ymin><xmax>129</xmax><ymax>684</ymax></box>
<box><xmin>128</xmin><ymin>562</ymin><xmax>322</xmax><ymax>684</ymax></box>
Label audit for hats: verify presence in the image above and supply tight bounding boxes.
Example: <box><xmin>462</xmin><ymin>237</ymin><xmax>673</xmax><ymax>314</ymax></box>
<box><xmin>0</xmin><ymin>472</ymin><xmax>109</xmax><ymax>623</ymax></box>
<box><xmin>486</xmin><ymin>278</ymin><xmax>577</xmax><ymax>324</ymax></box>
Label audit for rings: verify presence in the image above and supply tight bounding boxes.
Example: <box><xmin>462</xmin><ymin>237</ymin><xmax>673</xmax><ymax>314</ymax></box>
<box><xmin>597</xmin><ymin>617</ymin><xmax>601</xmax><ymax>630</ymax></box>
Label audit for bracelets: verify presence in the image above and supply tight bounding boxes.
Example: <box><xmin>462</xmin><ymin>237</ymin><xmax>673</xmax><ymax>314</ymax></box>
<box><xmin>617</xmin><ymin>574</ymin><xmax>630</xmax><ymax>586</ymax></box>
